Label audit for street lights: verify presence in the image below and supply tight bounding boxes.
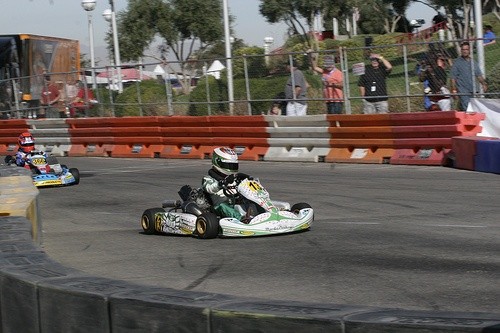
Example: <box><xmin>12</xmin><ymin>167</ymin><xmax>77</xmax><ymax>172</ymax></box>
<box><xmin>102</xmin><ymin>9</ymin><xmax>114</xmax><ymax>87</ymax></box>
<box><xmin>81</xmin><ymin>0</ymin><xmax>98</xmax><ymax>91</ymax></box>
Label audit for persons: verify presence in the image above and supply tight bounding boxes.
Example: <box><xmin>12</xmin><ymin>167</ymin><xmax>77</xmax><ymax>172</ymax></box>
<box><xmin>68</xmin><ymin>80</ymin><xmax>95</xmax><ymax>117</ymax></box>
<box><xmin>417</xmin><ymin>12</ymin><xmax>453</xmax><ymax>111</ymax></box>
<box><xmin>202</xmin><ymin>145</ymin><xmax>265</xmax><ymax>224</ymax></box>
<box><xmin>284</xmin><ymin>59</ymin><xmax>310</xmax><ymax>116</ymax></box>
<box><xmin>449</xmin><ymin>41</ymin><xmax>488</xmax><ymax>112</ymax></box>
<box><xmin>306</xmin><ymin>49</ymin><xmax>344</xmax><ymax>114</ymax></box>
<box><xmin>267</xmin><ymin>103</ymin><xmax>282</xmax><ymax>115</ymax></box>
<box><xmin>15</xmin><ymin>132</ymin><xmax>46</xmax><ymax>166</ymax></box>
<box><xmin>483</xmin><ymin>25</ymin><xmax>497</xmax><ymax>46</ymax></box>
<box><xmin>41</xmin><ymin>76</ymin><xmax>61</xmax><ymax>108</ymax></box>
<box><xmin>357</xmin><ymin>53</ymin><xmax>392</xmax><ymax>115</ymax></box>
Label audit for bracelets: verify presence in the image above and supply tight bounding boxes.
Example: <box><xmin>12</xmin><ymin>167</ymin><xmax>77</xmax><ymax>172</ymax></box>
<box><xmin>481</xmin><ymin>80</ymin><xmax>487</xmax><ymax>85</ymax></box>
<box><xmin>452</xmin><ymin>90</ymin><xmax>457</xmax><ymax>92</ymax></box>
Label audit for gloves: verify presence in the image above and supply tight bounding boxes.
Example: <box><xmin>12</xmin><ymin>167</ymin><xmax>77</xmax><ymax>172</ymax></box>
<box><xmin>236</xmin><ymin>172</ymin><xmax>249</xmax><ymax>182</ymax></box>
<box><xmin>221</xmin><ymin>174</ymin><xmax>235</xmax><ymax>186</ymax></box>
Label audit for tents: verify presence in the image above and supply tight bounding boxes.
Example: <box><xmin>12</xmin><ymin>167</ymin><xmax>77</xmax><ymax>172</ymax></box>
<box><xmin>93</xmin><ymin>68</ymin><xmax>155</xmax><ymax>84</ymax></box>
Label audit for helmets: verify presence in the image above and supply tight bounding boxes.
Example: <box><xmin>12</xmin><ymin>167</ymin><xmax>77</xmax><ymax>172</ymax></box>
<box><xmin>212</xmin><ymin>146</ymin><xmax>239</xmax><ymax>176</ymax></box>
<box><xmin>17</xmin><ymin>133</ymin><xmax>34</xmax><ymax>152</ymax></box>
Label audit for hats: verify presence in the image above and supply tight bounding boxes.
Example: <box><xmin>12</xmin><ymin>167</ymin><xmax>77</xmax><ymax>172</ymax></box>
<box><xmin>284</xmin><ymin>60</ymin><xmax>298</xmax><ymax>67</ymax></box>
<box><xmin>484</xmin><ymin>25</ymin><xmax>493</xmax><ymax>29</ymax></box>
